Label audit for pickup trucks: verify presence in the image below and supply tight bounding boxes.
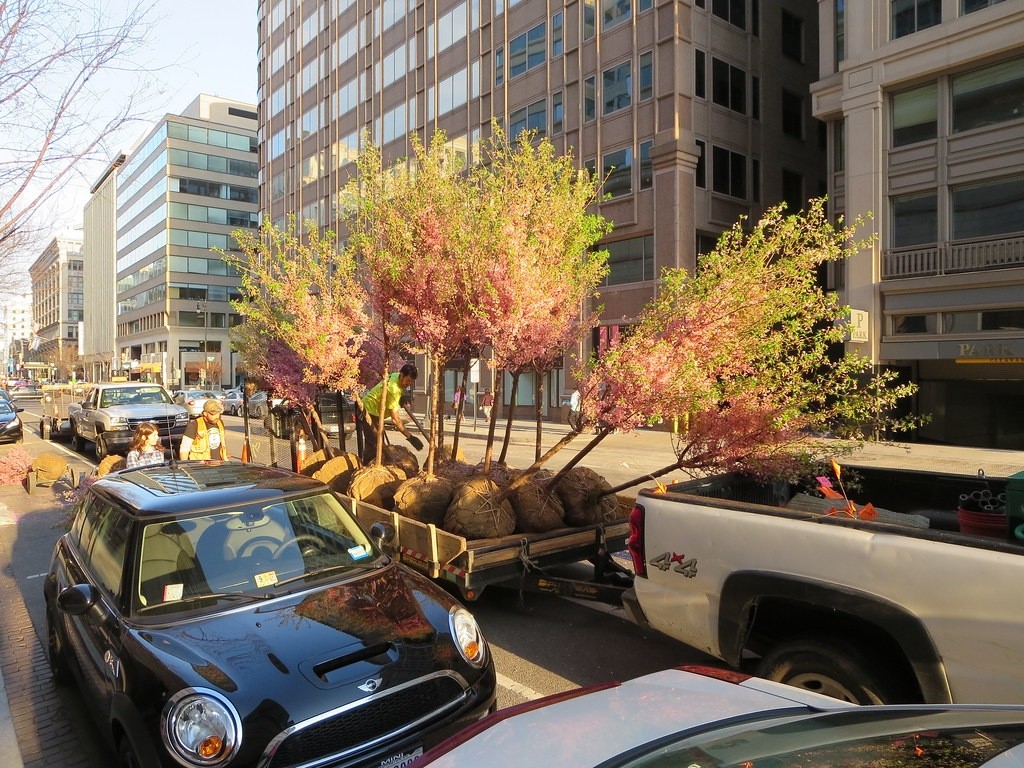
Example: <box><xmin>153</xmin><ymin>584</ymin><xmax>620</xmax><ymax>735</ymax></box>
<box><xmin>620</xmin><ymin>447</ymin><xmax>1024</xmax><ymax>713</ymax></box>
<box><xmin>68</xmin><ymin>382</ymin><xmax>191</xmax><ymax>466</ymax></box>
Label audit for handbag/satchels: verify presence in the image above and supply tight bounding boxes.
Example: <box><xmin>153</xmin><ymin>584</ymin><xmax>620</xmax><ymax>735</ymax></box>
<box><xmin>452</xmin><ymin>402</ymin><xmax>458</xmax><ymax>409</ymax></box>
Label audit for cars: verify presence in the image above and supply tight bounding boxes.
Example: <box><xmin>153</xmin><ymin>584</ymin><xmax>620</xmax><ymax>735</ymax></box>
<box><xmin>8</xmin><ymin>385</ymin><xmax>60</xmax><ymax>401</ymax></box>
<box><xmin>0</xmin><ymin>399</ymin><xmax>25</xmax><ymax>445</ymax></box>
<box><xmin>170</xmin><ymin>390</ymin><xmax>225</xmax><ymax>418</ymax></box>
<box><xmin>378</xmin><ymin>661</ymin><xmax>1024</xmax><ymax>767</ymax></box>
<box><xmin>0</xmin><ymin>389</ymin><xmax>18</xmax><ymax>405</ymax></box>
<box><xmin>220</xmin><ymin>383</ymin><xmax>359</xmax><ymax>442</ymax></box>
<box><xmin>43</xmin><ymin>456</ymin><xmax>503</xmax><ymax>768</ymax></box>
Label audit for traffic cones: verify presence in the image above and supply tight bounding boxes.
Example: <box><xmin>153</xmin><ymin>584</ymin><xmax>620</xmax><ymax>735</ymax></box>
<box><xmin>296</xmin><ymin>429</ymin><xmax>307</xmax><ymax>476</ymax></box>
<box><xmin>240</xmin><ymin>437</ymin><xmax>252</xmax><ymax>465</ymax></box>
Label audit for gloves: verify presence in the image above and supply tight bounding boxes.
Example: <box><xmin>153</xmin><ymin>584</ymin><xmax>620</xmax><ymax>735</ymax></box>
<box><xmin>406</xmin><ymin>435</ymin><xmax>424</xmax><ymax>452</ymax></box>
<box><xmin>399</xmin><ymin>395</ymin><xmax>407</xmax><ymax>408</ymax></box>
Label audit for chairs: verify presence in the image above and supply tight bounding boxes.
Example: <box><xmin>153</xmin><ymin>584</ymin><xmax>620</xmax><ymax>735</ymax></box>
<box><xmin>113</xmin><ymin>523</ymin><xmax>194</xmax><ymax>583</ymax></box>
<box><xmin>139</xmin><ymin>393</ymin><xmax>151</xmax><ymax>399</ymax></box>
<box><xmin>104</xmin><ymin>396</ymin><xmax>113</xmax><ymax>406</ymax></box>
<box><xmin>223</xmin><ymin>520</ymin><xmax>286</xmax><ymax>560</ymax></box>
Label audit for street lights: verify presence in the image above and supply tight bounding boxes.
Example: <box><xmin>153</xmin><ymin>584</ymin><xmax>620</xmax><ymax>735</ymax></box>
<box><xmin>196</xmin><ymin>298</ymin><xmax>209</xmax><ymax>390</ymax></box>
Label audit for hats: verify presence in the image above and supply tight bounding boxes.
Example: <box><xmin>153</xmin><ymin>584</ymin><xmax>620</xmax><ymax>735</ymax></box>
<box><xmin>203</xmin><ymin>400</ymin><xmax>222</xmax><ymax>415</ymax></box>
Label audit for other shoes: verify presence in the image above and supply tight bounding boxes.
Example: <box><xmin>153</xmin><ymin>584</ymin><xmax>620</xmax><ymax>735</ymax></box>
<box><xmin>485</xmin><ymin>416</ymin><xmax>491</xmax><ymax>422</ymax></box>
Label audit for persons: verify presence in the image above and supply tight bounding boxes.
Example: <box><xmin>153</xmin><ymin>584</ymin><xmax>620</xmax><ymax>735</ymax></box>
<box><xmin>454</xmin><ymin>387</ymin><xmax>466</xmax><ymax>422</ymax></box>
<box><xmin>196</xmin><ymin>384</ymin><xmax>200</xmax><ymax>390</ymax></box>
<box><xmin>179</xmin><ymin>400</ymin><xmax>228</xmax><ymax>460</ymax></box>
<box><xmin>567</xmin><ymin>387</ymin><xmax>580</xmax><ymax>430</ymax></box>
<box><xmin>480</xmin><ymin>389</ymin><xmax>492</xmax><ymax>422</ymax></box>
<box><xmin>358</xmin><ymin>366</ymin><xmax>424</xmax><ymax>465</ymax></box>
<box><xmin>126</xmin><ymin>423</ymin><xmax>165</xmax><ymax>469</ymax></box>
<box><xmin>592</xmin><ymin>423</ymin><xmax>603</xmax><ymax>434</ymax></box>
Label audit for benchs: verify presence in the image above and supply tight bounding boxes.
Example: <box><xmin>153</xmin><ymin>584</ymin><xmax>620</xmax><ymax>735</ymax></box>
<box><xmin>116</xmin><ymin>512</ymin><xmax>242</xmax><ymax>566</ymax></box>
